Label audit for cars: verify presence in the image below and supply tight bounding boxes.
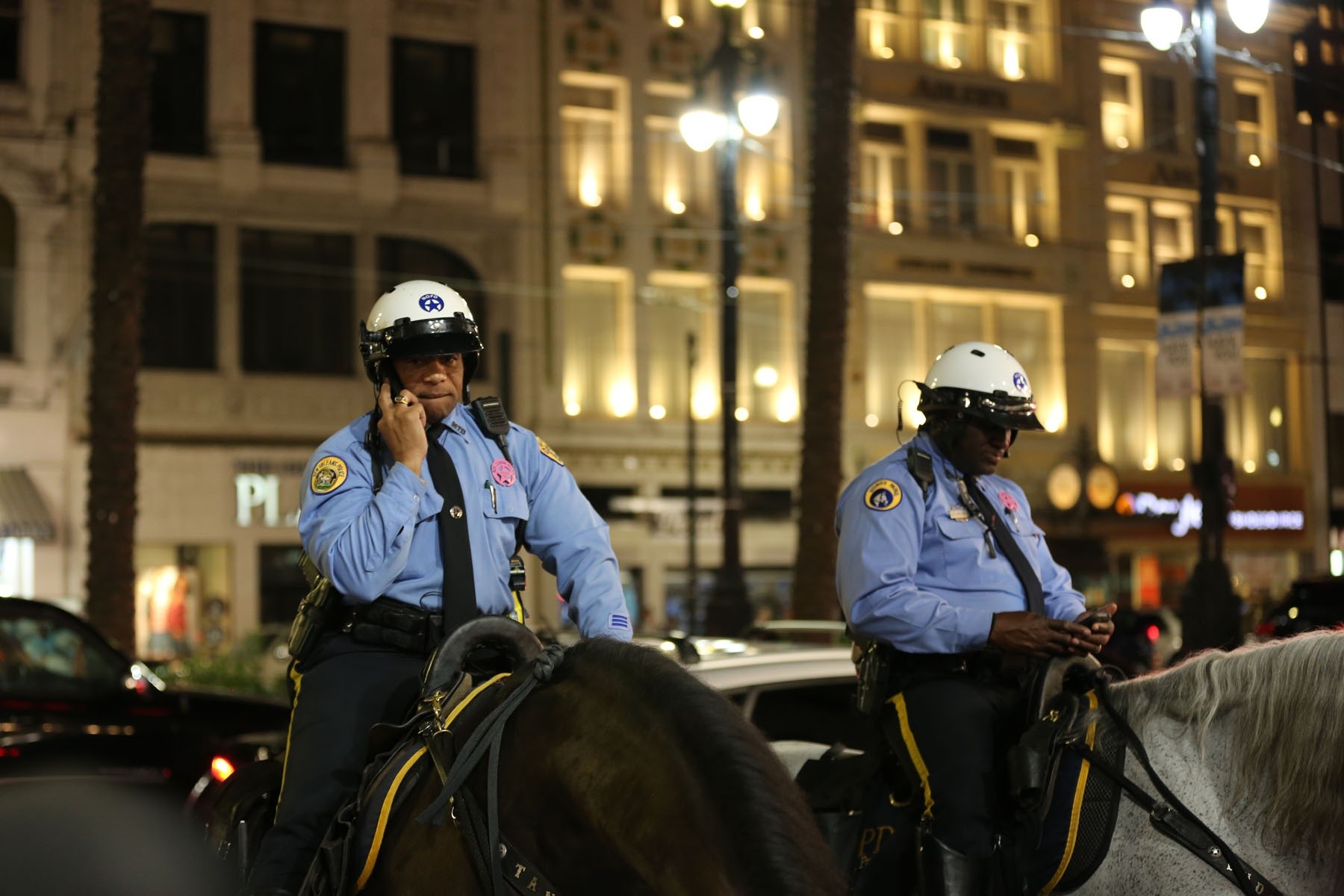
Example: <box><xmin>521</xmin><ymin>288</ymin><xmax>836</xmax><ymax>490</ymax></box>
<box><xmin>0</xmin><ymin>595</ymin><xmax>291</xmax><ymax>895</ymax></box>
<box><xmin>630</xmin><ymin>618</ymin><xmax>868</xmax><ymax>778</ymax></box>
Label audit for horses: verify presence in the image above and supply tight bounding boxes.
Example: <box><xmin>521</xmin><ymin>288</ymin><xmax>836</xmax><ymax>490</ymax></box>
<box><xmin>296</xmin><ymin>625</ymin><xmax>1344</xmax><ymax>896</ymax></box>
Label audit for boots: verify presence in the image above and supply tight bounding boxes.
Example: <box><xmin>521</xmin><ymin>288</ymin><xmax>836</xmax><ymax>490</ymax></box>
<box><xmin>929</xmin><ymin>830</ymin><xmax>1001</xmax><ymax>896</ymax></box>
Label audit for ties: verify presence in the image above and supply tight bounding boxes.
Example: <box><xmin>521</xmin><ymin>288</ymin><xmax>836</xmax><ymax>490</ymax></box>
<box><xmin>963</xmin><ymin>476</ymin><xmax>1045</xmax><ymax>615</ymax></box>
<box><xmin>426</xmin><ymin>422</ymin><xmax>477</xmax><ymax>638</ymax></box>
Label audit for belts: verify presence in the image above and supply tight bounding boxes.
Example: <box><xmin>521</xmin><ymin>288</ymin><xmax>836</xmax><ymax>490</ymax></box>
<box><xmin>358</xmin><ymin>604</ymin><xmax>430</xmax><ymax>636</ymax></box>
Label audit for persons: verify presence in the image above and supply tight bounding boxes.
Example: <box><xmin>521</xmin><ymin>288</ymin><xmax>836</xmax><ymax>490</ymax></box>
<box><xmin>834</xmin><ymin>341</ymin><xmax>1117</xmax><ymax>896</ymax></box>
<box><xmin>244</xmin><ymin>278</ymin><xmax>635</xmax><ymax>896</ymax></box>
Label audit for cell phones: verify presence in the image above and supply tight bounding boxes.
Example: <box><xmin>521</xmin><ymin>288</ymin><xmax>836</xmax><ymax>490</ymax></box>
<box><xmin>1071</xmin><ymin>612</ymin><xmax>1112</xmax><ymax>634</ymax></box>
<box><xmin>377</xmin><ymin>360</ymin><xmax>406</xmax><ymax>404</ymax></box>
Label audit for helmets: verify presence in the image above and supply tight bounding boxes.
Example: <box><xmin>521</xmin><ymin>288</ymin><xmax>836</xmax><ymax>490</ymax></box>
<box><xmin>917</xmin><ymin>341</ymin><xmax>1045</xmax><ymax>432</ymax></box>
<box><xmin>359</xmin><ymin>278</ymin><xmax>484</xmax><ymax>388</ymax></box>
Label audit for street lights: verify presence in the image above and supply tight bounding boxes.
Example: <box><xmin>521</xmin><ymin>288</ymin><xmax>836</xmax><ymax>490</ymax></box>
<box><xmin>677</xmin><ymin>0</ymin><xmax>784</xmax><ymax>638</ymax></box>
<box><xmin>1138</xmin><ymin>0</ymin><xmax>1286</xmax><ymax>667</ymax></box>
